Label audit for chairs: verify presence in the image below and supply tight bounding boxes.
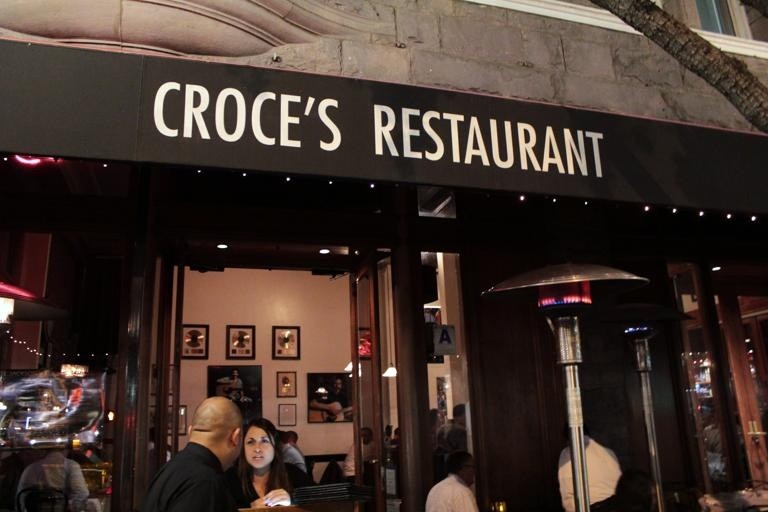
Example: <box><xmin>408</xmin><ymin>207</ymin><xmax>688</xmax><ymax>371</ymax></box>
<box><xmin>292</xmin><ymin>482</ymin><xmax>366</xmax><ymax>512</ymax></box>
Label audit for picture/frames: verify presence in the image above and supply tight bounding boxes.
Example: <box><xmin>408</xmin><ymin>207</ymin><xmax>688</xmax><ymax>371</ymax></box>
<box><xmin>226</xmin><ymin>325</ymin><xmax>255</xmax><ymax>360</ymax></box>
<box><xmin>276</xmin><ymin>371</ymin><xmax>297</xmax><ymax>398</ymax></box>
<box><xmin>272</xmin><ymin>326</ymin><xmax>300</xmax><ymax>360</ymax></box>
<box><xmin>180</xmin><ymin>324</ymin><xmax>209</xmax><ymax>360</ymax></box>
<box><xmin>207</xmin><ymin>365</ymin><xmax>262</xmax><ymax>428</ymax></box>
<box><xmin>279</xmin><ymin>404</ymin><xmax>296</xmax><ymax>426</ymax></box>
<box><xmin>149</xmin><ymin>405</ymin><xmax>187</xmax><ymax>436</ymax></box>
<box><xmin>150</xmin><ymin>363</ymin><xmax>174</xmax><ymax>396</ymax></box>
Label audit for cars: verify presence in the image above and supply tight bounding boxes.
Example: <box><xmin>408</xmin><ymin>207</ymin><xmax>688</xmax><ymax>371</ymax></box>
<box><xmin>0</xmin><ymin>426</ymin><xmax>82</xmax><ymax>451</ymax></box>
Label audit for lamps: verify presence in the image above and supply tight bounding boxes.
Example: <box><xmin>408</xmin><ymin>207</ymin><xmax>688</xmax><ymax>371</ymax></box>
<box><xmin>382</xmin><ymin>263</ymin><xmax>398</xmax><ymax>377</ymax></box>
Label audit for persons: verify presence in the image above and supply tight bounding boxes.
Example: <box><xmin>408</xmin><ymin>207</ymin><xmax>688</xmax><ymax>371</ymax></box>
<box><xmin>216</xmin><ymin>369</ymin><xmax>253</xmax><ymax>416</ymax></box>
<box><xmin>558</xmin><ymin>418</ymin><xmax>625</xmax><ymax>512</ymax></box>
<box><xmin>223</xmin><ymin>418</ymin><xmax>320</xmax><ymax>510</ymax></box>
<box><xmin>136</xmin><ymin>397</ymin><xmax>245</xmax><ymax>511</ymax></box>
<box><xmin>14</xmin><ymin>437</ymin><xmax>90</xmax><ymax>512</ymax></box>
<box><xmin>341</xmin><ymin>403</ymin><xmax>480</xmax><ymax>512</ymax></box>
